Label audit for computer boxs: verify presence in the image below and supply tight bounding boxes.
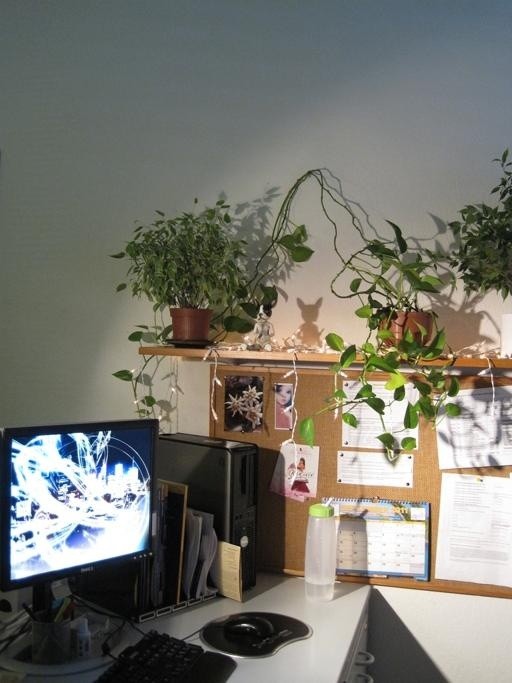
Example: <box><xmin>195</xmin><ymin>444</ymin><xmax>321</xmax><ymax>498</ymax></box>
<box><xmin>158</xmin><ymin>433</ymin><xmax>257</xmax><ymax>592</ymax></box>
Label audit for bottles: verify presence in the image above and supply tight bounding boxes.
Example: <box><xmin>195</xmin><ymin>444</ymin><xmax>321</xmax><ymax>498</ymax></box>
<box><xmin>76</xmin><ymin>617</ymin><xmax>92</xmax><ymax>658</ymax></box>
<box><xmin>306</xmin><ymin>504</ymin><xmax>335</xmax><ymax>603</ymax></box>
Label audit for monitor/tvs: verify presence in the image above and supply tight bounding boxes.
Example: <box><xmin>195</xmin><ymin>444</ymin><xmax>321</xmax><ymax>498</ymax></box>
<box><xmin>0</xmin><ymin>419</ymin><xmax>159</xmax><ymax>676</ymax></box>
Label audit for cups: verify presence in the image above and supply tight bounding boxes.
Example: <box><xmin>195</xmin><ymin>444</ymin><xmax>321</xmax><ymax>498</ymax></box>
<box><xmin>30</xmin><ymin>609</ymin><xmax>71</xmax><ymax>664</ymax></box>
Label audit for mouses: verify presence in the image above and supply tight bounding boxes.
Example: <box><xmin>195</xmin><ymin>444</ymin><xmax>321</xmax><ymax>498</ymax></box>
<box><xmin>225</xmin><ymin>618</ymin><xmax>273</xmax><ymax>638</ymax></box>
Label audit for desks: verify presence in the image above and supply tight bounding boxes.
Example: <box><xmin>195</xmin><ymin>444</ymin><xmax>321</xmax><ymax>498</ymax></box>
<box><xmin>0</xmin><ymin>572</ymin><xmax>375</xmax><ymax>683</ymax></box>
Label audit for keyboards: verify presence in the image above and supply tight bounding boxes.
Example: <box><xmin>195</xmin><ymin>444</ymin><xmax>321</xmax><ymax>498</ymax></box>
<box><xmin>93</xmin><ymin>630</ymin><xmax>237</xmax><ymax>683</ymax></box>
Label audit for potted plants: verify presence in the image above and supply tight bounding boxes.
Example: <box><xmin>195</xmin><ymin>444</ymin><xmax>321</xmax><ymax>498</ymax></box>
<box><xmin>297</xmin><ymin>217</ymin><xmax>458</xmax><ymax>453</ymax></box>
<box><xmin>110</xmin><ymin>195</ymin><xmax>253</xmax><ymax>345</ymax></box>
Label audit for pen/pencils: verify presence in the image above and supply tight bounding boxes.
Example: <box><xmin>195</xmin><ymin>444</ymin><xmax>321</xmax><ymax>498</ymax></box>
<box><xmin>20</xmin><ymin>593</ymin><xmax>75</xmax><ymax>663</ymax></box>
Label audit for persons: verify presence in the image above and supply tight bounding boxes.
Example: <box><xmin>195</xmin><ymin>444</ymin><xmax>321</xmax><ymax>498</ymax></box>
<box><xmin>275</xmin><ymin>386</ymin><xmax>291</xmax><ymax>428</ymax></box>
<box><xmin>291</xmin><ymin>459</ymin><xmax>312</xmax><ymax>495</ymax></box>
